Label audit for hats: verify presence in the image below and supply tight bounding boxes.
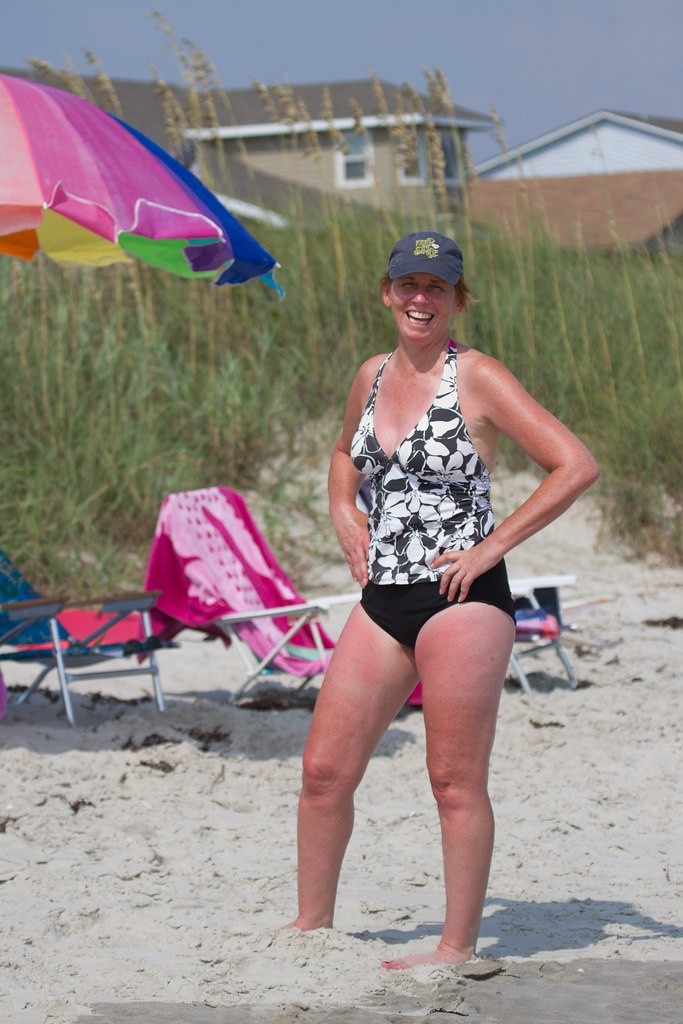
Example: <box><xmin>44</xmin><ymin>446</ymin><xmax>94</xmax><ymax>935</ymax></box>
<box><xmin>388</xmin><ymin>232</ymin><xmax>464</xmax><ymax>284</ymax></box>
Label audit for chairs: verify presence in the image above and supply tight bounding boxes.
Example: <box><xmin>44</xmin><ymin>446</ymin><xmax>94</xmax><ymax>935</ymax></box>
<box><xmin>0</xmin><ymin>549</ymin><xmax>162</xmax><ymax>727</ymax></box>
<box><xmin>146</xmin><ymin>486</ymin><xmax>582</xmax><ymax>706</ymax></box>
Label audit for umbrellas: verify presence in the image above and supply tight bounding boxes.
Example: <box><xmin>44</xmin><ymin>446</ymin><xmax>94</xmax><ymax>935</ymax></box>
<box><xmin>0</xmin><ymin>68</ymin><xmax>277</xmax><ymax>288</ymax></box>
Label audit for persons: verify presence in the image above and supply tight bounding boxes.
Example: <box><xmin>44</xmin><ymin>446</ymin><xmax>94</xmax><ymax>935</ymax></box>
<box><xmin>280</xmin><ymin>229</ymin><xmax>600</xmax><ymax>970</ymax></box>
